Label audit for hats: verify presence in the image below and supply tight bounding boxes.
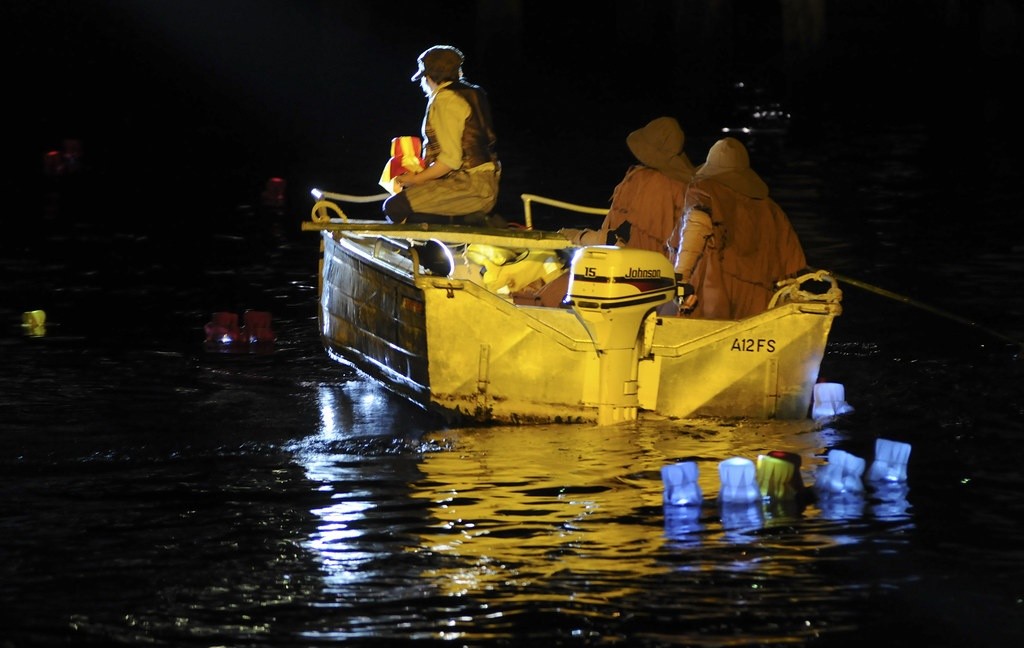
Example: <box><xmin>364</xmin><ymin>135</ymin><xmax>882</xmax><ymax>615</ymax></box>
<box><xmin>696</xmin><ymin>138</ymin><xmax>768</xmax><ymax>199</ymax></box>
<box><xmin>626</xmin><ymin>117</ymin><xmax>697</xmax><ymax>183</ymax></box>
<box><xmin>410</xmin><ymin>44</ymin><xmax>465</xmax><ymax>82</ymax></box>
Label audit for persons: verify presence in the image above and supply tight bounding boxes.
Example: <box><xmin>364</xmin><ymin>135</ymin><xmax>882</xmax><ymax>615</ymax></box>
<box><xmin>558</xmin><ymin>118</ymin><xmax>693</xmax><ymax>262</ymax></box>
<box><xmin>382</xmin><ymin>46</ymin><xmax>501</xmax><ymax>225</ymax></box>
<box><xmin>674</xmin><ymin>137</ymin><xmax>806</xmax><ymax>320</ymax></box>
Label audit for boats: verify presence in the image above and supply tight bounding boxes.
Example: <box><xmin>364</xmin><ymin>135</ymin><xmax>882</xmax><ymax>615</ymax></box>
<box><xmin>300</xmin><ymin>195</ymin><xmax>848</xmax><ymax>420</ymax></box>
